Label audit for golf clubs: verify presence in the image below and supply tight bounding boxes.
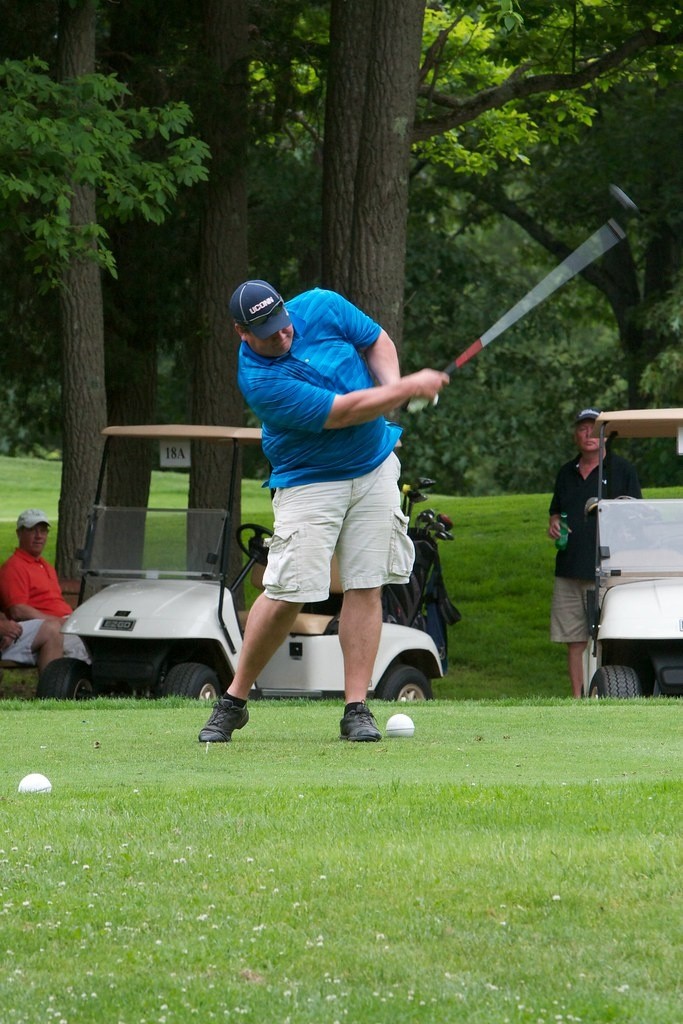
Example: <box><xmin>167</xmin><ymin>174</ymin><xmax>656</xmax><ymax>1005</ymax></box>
<box><xmin>399</xmin><ymin>180</ymin><xmax>646</xmax><ymax>414</ymax></box>
<box><xmin>414</xmin><ymin>508</ymin><xmax>455</xmax><ymax>545</ymax></box>
<box><xmin>401</xmin><ymin>476</ymin><xmax>438</xmax><ymax>535</ymax></box>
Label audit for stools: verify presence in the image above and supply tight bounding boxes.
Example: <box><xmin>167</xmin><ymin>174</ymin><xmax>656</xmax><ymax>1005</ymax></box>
<box><xmin>0</xmin><ymin>660</ymin><xmax>37</xmax><ymax>668</ymax></box>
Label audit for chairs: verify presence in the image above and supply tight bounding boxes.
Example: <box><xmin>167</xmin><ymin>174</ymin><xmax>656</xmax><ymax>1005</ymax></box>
<box><xmin>595</xmin><ymin>549</ymin><xmax>683</xmax><ymax>608</ymax></box>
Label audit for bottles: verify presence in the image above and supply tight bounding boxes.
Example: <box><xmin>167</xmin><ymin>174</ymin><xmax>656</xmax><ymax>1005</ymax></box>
<box><xmin>554</xmin><ymin>512</ymin><xmax>569</xmax><ymax>551</ymax></box>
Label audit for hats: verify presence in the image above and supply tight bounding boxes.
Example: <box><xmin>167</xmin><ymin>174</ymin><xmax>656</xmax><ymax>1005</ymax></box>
<box><xmin>229</xmin><ymin>280</ymin><xmax>291</xmax><ymax>339</ymax></box>
<box><xmin>575</xmin><ymin>408</ymin><xmax>607</xmax><ymax>424</ymax></box>
<box><xmin>17</xmin><ymin>509</ymin><xmax>51</xmax><ymax>530</ymax></box>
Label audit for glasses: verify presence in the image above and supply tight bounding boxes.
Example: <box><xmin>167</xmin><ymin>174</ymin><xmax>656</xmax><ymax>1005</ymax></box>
<box><xmin>235</xmin><ymin>295</ymin><xmax>284</xmax><ymax>329</ymax></box>
<box><xmin>24</xmin><ymin>528</ymin><xmax>49</xmax><ymax>535</ymax></box>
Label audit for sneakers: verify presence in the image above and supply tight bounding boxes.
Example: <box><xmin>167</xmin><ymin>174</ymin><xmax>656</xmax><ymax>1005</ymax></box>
<box><xmin>198</xmin><ymin>696</ymin><xmax>249</xmax><ymax>743</ymax></box>
<box><xmin>340</xmin><ymin>700</ymin><xmax>382</xmax><ymax>743</ymax></box>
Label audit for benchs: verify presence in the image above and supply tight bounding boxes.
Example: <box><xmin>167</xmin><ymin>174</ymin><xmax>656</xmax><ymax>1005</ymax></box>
<box><xmin>236</xmin><ymin>551</ymin><xmax>343</xmax><ymax>636</ymax></box>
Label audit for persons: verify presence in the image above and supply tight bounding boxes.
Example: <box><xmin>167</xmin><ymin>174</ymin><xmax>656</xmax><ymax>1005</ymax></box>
<box><xmin>0</xmin><ymin>509</ymin><xmax>90</xmax><ymax>675</ymax></box>
<box><xmin>198</xmin><ymin>280</ymin><xmax>449</xmax><ymax>742</ymax></box>
<box><xmin>547</xmin><ymin>408</ymin><xmax>642</xmax><ymax>699</ymax></box>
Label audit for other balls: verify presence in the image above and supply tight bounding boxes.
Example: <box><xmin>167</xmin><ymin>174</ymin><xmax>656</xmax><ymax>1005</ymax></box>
<box><xmin>386</xmin><ymin>713</ymin><xmax>415</xmax><ymax>739</ymax></box>
<box><xmin>17</xmin><ymin>772</ymin><xmax>52</xmax><ymax>795</ymax></box>
